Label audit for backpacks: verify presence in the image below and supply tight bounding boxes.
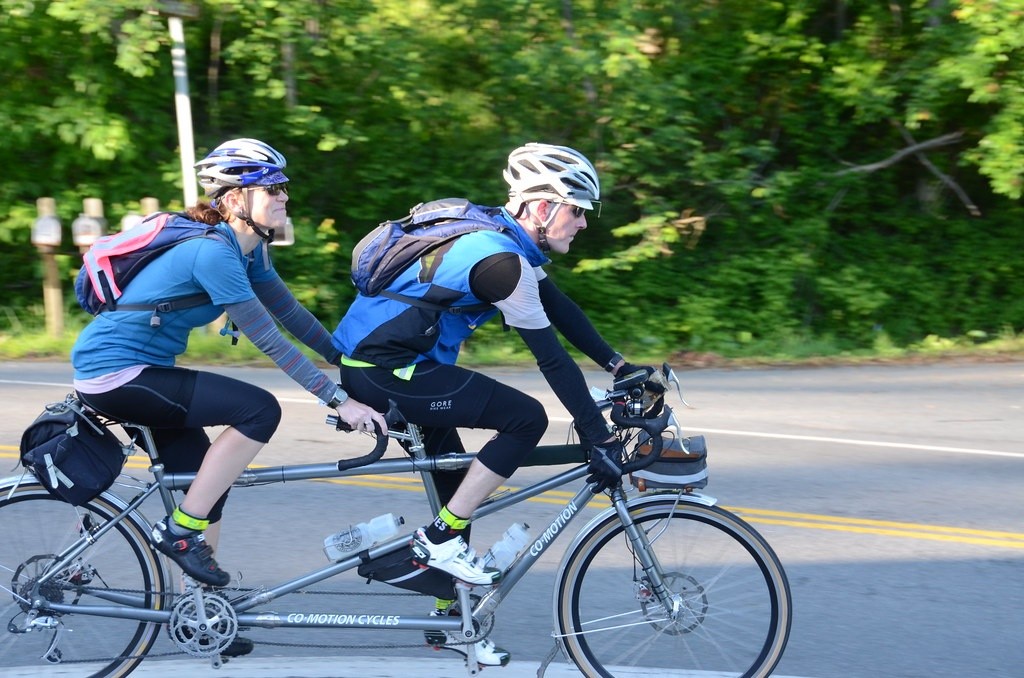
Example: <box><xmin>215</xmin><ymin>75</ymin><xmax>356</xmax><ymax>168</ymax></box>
<box><xmin>351</xmin><ymin>196</ymin><xmax>510</xmax><ymax>332</ymax></box>
<box><xmin>74</xmin><ymin>212</ymin><xmax>239</xmax><ymax>345</ymax></box>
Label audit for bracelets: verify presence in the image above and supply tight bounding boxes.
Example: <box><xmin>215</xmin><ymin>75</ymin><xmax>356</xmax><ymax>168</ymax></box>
<box><xmin>605</xmin><ymin>352</ymin><xmax>623</xmax><ymax>372</ymax></box>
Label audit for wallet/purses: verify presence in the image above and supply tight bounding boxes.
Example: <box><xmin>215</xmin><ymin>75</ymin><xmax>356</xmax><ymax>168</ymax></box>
<box><xmin>628</xmin><ymin>431</ymin><xmax>709</xmax><ymax>490</ymax></box>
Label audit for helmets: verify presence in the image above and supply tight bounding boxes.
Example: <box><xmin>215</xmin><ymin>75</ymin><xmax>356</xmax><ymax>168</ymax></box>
<box><xmin>192</xmin><ymin>138</ymin><xmax>290</xmax><ymax>199</ymax></box>
<box><xmin>503</xmin><ymin>143</ymin><xmax>600</xmax><ymax>219</ymax></box>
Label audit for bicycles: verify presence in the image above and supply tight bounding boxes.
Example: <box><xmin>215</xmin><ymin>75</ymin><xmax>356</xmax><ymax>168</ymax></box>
<box><xmin>1</xmin><ymin>365</ymin><xmax>797</xmax><ymax>678</ymax></box>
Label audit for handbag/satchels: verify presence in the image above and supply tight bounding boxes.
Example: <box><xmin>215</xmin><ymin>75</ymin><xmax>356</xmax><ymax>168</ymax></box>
<box><xmin>19</xmin><ymin>398</ymin><xmax>126</xmax><ymax>506</ymax></box>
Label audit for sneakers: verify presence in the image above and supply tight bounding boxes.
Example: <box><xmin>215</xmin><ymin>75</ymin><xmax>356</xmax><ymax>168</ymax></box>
<box><xmin>166</xmin><ymin>621</ymin><xmax>253</xmax><ymax>657</ymax></box>
<box><xmin>423</xmin><ymin>610</ymin><xmax>510</xmax><ymax>666</ymax></box>
<box><xmin>411</xmin><ymin>527</ymin><xmax>503</xmax><ymax>586</ymax></box>
<box><xmin>151</xmin><ymin>516</ymin><xmax>230</xmax><ymax>586</ymax></box>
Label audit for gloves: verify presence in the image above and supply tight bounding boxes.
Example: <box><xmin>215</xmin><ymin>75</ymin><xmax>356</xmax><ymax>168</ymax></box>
<box><xmin>585</xmin><ymin>438</ymin><xmax>623</xmax><ymax>494</ymax></box>
<box><xmin>613</xmin><ymin>361</ymin><xmax>665</xmax><ymax>394</ymax></box>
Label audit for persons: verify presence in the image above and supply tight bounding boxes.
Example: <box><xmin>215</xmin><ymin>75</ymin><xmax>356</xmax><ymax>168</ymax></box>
<box><xmin>330</xmin><ymin>141</ymin><xmax>655</xmax><ymax>667</ymax></box>
<box><xmin>72</xmin><ymin>138</ymin><xmax>389</xmax><ymax>656</ymax></box>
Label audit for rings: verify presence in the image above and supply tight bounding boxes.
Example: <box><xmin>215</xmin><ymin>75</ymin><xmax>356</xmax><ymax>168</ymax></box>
<box><xmin>365</xmin><ymin>421</ymin><xmax>371</xmax><ymax>424</ymax></box>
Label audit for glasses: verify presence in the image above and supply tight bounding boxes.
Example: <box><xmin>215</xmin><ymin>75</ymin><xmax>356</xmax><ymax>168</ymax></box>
<box><xmin>247</xmin><ymin>182</ymin><xmax>288</xmax><ymax>196</ymax></box>
<box><xmin>574</xmin><ymin>207</ymin><xmax>584</xmax><ymax>217</ymax></box>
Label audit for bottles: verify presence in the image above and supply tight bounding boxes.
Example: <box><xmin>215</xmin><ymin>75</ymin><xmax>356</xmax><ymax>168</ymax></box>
<box><xmin>480</xmin><ymin>521</ymin><xmax>532</xmax><ymax>590</ymax></box>
<box><xmin>322</xmin><ymin>512</ymin><xmax>405</xmax><ymax>563</ymax></box>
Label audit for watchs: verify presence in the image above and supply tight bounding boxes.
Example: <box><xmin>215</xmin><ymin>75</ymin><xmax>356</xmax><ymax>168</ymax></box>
<box><xmin>327</xmin><ymin>388</ymin><xmax>348</xmax><ymax>409</ymax></box>
